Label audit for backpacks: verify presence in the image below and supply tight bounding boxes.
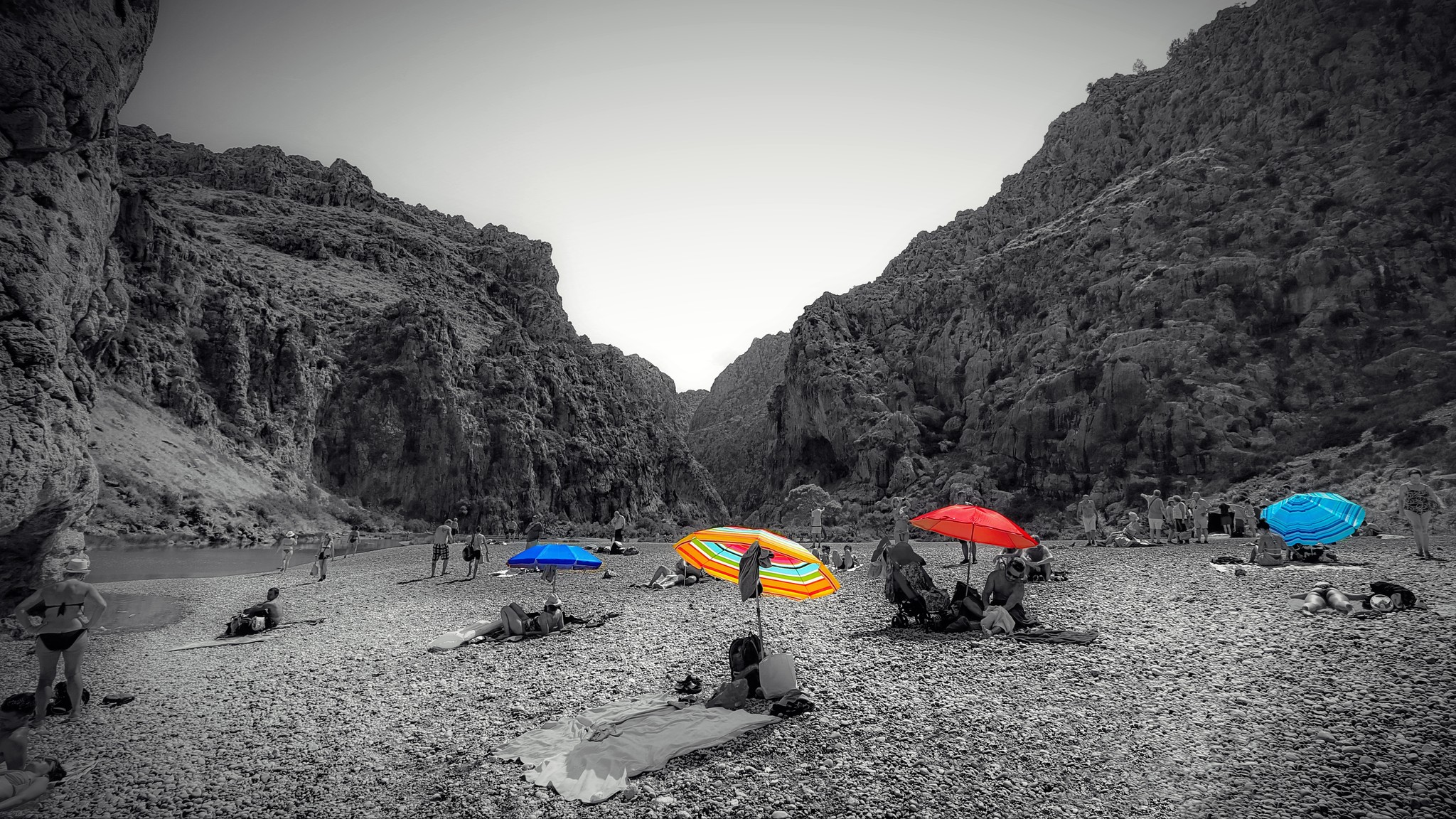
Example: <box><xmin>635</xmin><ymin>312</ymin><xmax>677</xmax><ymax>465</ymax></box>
<box><xmin>462</xmin><ymin>534</ymin><xmax>475</xmax><ymax>561</ymax></box>
<box><xmin>583</xmin><ymin>541</ymin><xmax>639</xmax><ymax>556</ymax></box>
<box><xmin>707</xmin><ymin>631</ymin><xmax>766</xmax><ymax>711</ymax></box>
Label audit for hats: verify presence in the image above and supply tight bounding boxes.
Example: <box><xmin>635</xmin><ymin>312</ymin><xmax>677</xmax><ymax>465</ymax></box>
<box><xmin>61</xmin><ymin>558</ymin><xmax>92</xmax><ymax>573</ymax></box>
<box><xmin>545</xmin><ymin>593</ymin><xmax>562</xmax><ymax>606</ymax></box>
<box><xmin>887</xmin><ymin>541</ymin><xmax>922</xmax><ymax>562</ymax></box>
<box><xmin>1167</xmin><ymin>497</ymin><xmax>1174</xmax><ymax>503</ymax></box>
<box><xmin>533</xmin><ymin>515</ymin><xmax>539</xmax><ymax>518</ymax></box>
<box><xmin>286</xmin><ymin>531</ymin><xmax>295</xmax><ymax>537</ymax></box>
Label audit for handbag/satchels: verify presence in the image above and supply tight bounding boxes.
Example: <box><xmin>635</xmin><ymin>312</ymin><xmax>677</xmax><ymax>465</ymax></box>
<box><xmin>478</xmin><ymin>556</ymin><xmax>484</xmax><ymax>564</ymax></box>
<box><xmin>759</xmin><ymin>643</ymin><xmax>797</xmax><ymax>699</ymax></box>
<box><xmin>1178</xmin><ymin>527</ymin><xmax>1192</xmax><ymax>540</ymax></box>
<box><xmin>868</xmin><ymin>543</ymin><xmax>887</xmax><ymax>579</ymax></box>
<box><xmin>318</xmin><ymin>552</ymin><xmax>324</xmax><ymax>560</ymax></box>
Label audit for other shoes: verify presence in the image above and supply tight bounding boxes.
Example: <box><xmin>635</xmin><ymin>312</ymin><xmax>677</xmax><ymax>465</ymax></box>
<box><xmin>960</xmin><ymin>559</ymin><xmax>978</xmax><ymax>564</ymax></box>
<box><xmin>442</xmin><ymin>572</ymin><xmax>451</xmax><ymax>575</ymax></box>
<box><xmin>431</xmin><ymin>575</ymin><xmax>438</xmax><ymax>578</ymax></box>
<box><xmin>486</xmin><ymin>554</ymin><xmax>490</xmax><ymax>562</ymax></box>
<box><xmin>1086</xmin><ymin>540</ymin><xmax>1208</xmax><ymax>547</ymax></box>
<box><xmin>317</xmin><ymin>575</ymin><xmax>326</xmax><ymax>582</ymax></box>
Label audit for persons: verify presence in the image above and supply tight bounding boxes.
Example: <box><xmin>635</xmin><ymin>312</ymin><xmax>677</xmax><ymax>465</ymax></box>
<box><xmin>276</xmin><ymin>531</ymin><xmax>298</xmax><ymax>573</ymax></box>
<box><xmin>343</xmin><ymin>525</ymin><xmax>361</xmax><ymax>558</ymax></box>
<box><xmin>242</xmin><ymin>588</ymin><xmax>283</xmax><ymax>629</ymax></box>
<box><xmin>1359</xmin><ymin>468</ymin><xmax>1449</xmax><ymax>558</ymax></box>
<box><xmin>1076</xmin><ymin>484</ymin><xmax>1306</xmax><ymax>566</ymax></box>
<box><xmin>315</xmin><ymin>532</ymin><xmax>334</xmax><ymax>583</ymax></box>
<box><xmin>431</xmin><ymin>515</ymin><xmax>544</xmax><ymax>580</ymax></box>
<box><xmin>609</xmin><ymin>511</ymin><xmax>630</xmax><ymax>543</ymax></box>
<box><xmin>892</xmin><ymin>496</ymin><xmax>911</xmax><ymax>543</ymax></box>
<box><xmin>496</xmin><ymin>593</ymin><xmax>564</xmax><ymax>640</ymax></box>
<box><xmin>407</xmin><ymin>530</ymin><xmax>410</xmax><ymax>541</ymax></box>
<box><xmin>0</xmin><ymin>559</ymin><xmax>107</xmax><ymax>810</ymax></box>
<box><xmin>809</xmin><ymin>502</ymin><xmax>860</xmax><ymax>573</ymax></box>
<box><xmin>955</xmin><ymin>491</ymin><xmax>1055</xmax><ymax>639</ymax></box>
<box><xmin>644</xmin><ymin>532</ymin><xmax>703</xmax><ymax>590</ymax></box>
<box><xmin>1289</xmin><ymin>581</ymin><xmax>1371</xmax><ymax>618</ymax></box>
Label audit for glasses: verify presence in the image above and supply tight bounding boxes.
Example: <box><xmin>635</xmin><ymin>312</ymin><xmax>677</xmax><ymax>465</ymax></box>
<box><xmin>822</xmin><ymin>549</ymin><xmax>826</xmax><ymax>551</ymax></box>
<box><xmin>31</xmin><ymin>757</ymin><xmax>56</xmax><ymax>773</ymax></box>
<box><xmin>1007</xmin><ymin>571</ymin><xmax>1019</xmax><ymax>579</ymax></box>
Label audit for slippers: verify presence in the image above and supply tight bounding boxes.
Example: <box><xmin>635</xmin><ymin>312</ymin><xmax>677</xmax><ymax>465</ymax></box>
<box><xmin>675</xmin><ymin>675</ymin><xmax>703</xmax><ymax>693</ymax></box>
<box><xmin>585</xmin><ymin>723</ymin><xmax>623</xmax><ymax>741</ymax></box>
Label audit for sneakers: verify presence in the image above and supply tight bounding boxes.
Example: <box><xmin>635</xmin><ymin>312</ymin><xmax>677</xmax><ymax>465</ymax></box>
<box><xmin>810</xmin><ymin>545</ymin><xmax>821</xmax><ymax>548</ymax></box>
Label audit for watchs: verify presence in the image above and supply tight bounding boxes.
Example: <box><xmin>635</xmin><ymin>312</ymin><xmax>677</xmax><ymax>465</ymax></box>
<box><xmin>1295</xmin><ymin>552</ymin><xmax>1298</xmax><ymax>555</ymax></box>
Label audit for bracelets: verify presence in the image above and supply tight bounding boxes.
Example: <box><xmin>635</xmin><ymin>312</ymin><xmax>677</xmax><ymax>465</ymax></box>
<box><xmin>315</xmin><ymin>555</ymin><xmax>318</xmax><ymax>557</ymax></box>
<box><xmin>1205</xmin><ymin>512</ymin><xmax>1207</xmax><ymax>514</ymax></box>
<box><xmin>1182</xmin><ymin>519</ymin><xmax>1184</xmax><ymax>520</ymax></box>
<box><xmin>1169</xmin><ymin>521</ymin><xmax>1172</xmax><ymax>522</ymax></box>
<box><xmin>1096</xmin><ymin>516</ymin><xmax>1099</xmax><ymax>517</ymax></box>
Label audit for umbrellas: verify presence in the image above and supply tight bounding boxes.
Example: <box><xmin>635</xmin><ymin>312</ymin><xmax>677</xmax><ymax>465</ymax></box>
<box><xmin>1259</xmin><ymin>492</ymin><xmax>1365</xmax><ymax>556</ymax></box>
<box><xmin>674</xmin><ymin>527</ymin><xmax>842</xmax><ymax>660</ymax></box>
<box><xmin>505</xmin><ymin>543</ymin><xmax>603</xmax><ymax>592</ymax></box>
<box><xmin>909</xmin><ymin>503</ymin><xmax>1039</xmax><ymax>597</ymax></box>
